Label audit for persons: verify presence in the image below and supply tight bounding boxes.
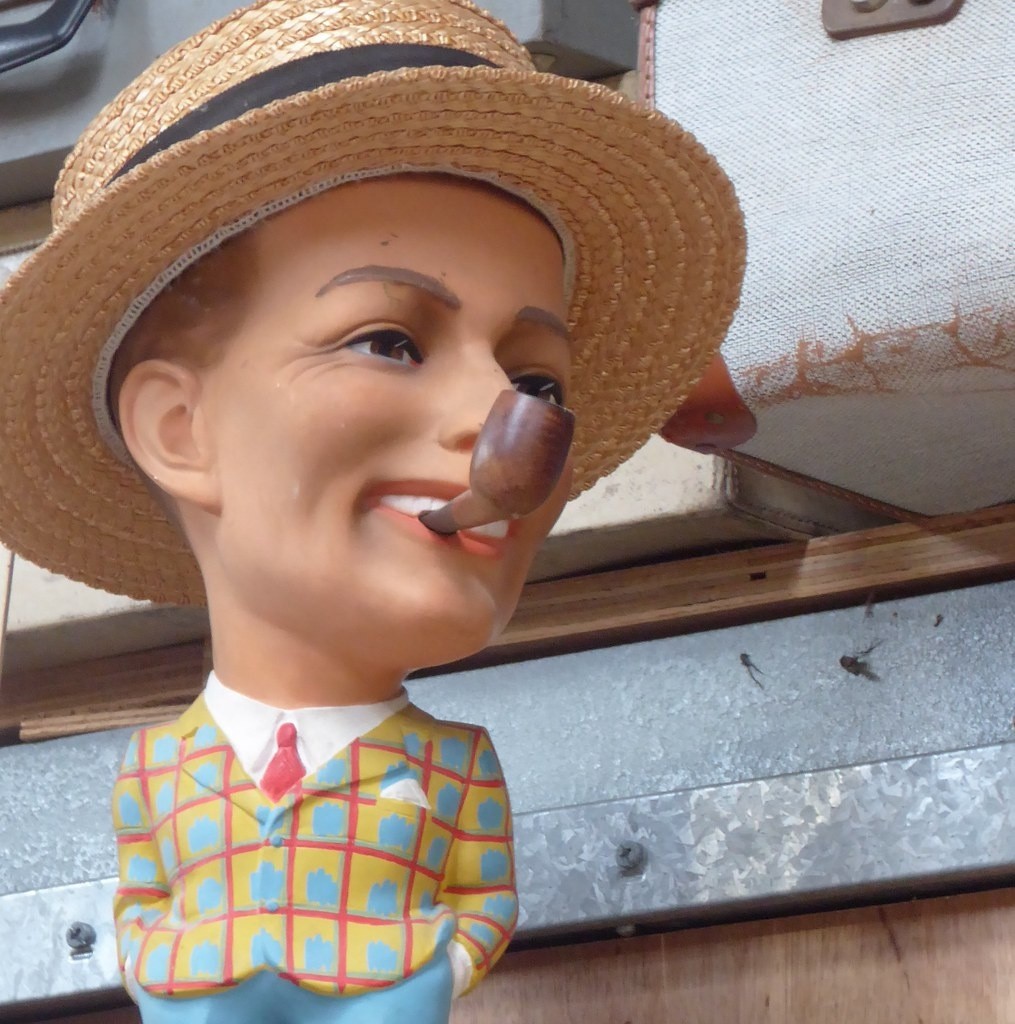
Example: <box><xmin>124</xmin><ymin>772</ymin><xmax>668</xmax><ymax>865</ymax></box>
<box><xmin>0</xmin><ymin>0</ymin><xmax>748</xmax><ymax>1024</ymax></box>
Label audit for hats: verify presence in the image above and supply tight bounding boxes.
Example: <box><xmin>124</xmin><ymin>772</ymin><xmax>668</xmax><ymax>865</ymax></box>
<box><xmin>0</xmin><ymin>0</ymin><xmax>752</xmax><ymax>606</ymax></box>
